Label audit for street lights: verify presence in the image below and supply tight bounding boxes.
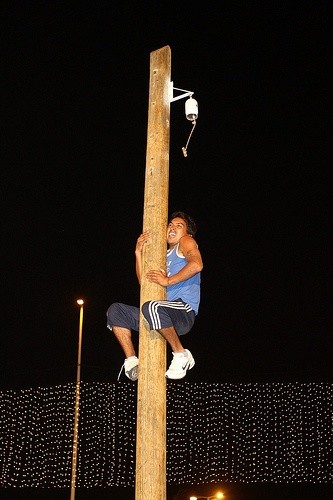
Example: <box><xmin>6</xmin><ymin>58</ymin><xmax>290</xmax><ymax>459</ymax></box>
<box><xmin>69</xmin><ymin>298</ymin><xmax>87</xmax><ymax>500</ymax></box>
<box><xmin>134</xmin><ymin>46</ymin><xmax>201</xmax><ymax>500</ymax></box>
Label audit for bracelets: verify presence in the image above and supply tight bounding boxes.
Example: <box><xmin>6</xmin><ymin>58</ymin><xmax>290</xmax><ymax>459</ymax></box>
<box><xmin>167</xmin><ymin>276</ymin><xmax>171</xmax><ymax>286</ymax></box>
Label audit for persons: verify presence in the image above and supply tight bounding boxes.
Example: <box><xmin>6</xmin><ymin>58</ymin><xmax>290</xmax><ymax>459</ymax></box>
<box><xmin>105</xmin><ymin>210</ymin><xmax>203</xmax><ymax>381</ymax></box>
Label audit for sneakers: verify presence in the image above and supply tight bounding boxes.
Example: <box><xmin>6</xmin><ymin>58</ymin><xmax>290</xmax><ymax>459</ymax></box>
<box><xmin>117</xmin><ymin>356</ymin><xmax>138</xmax><ymax>381</ymax></box>
<box><xmin>165</xmin><ymin>349</ymin><xmax>196</xmax><ymax>379</ymax></box>
